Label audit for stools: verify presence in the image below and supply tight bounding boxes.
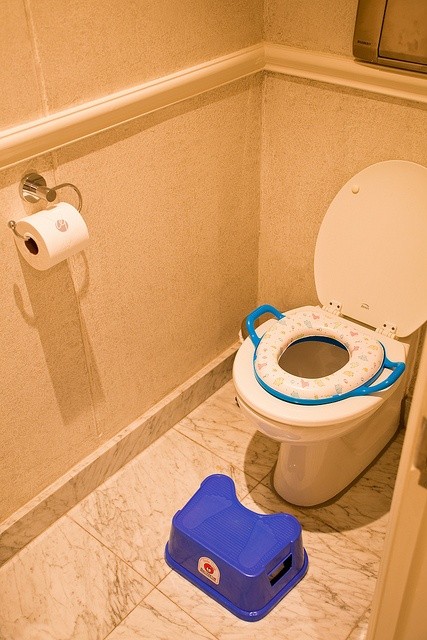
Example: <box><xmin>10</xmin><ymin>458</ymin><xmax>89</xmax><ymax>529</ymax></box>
<box><xmin>163</xmin><ymin>474</ymin><xmax>308</xmax><ymax>621</ymax></box>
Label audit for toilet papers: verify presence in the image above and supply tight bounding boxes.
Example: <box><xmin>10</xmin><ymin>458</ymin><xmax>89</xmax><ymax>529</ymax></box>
<box><xmin>13</xmin><ymin>202</ymin><xmax>89</xmax><ymax>271</ymax></box>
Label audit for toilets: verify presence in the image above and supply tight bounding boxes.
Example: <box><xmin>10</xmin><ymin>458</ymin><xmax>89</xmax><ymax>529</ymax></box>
<box><xmin>232</xmin><ymin>159</ymin><xmax>427</xmax><ymax>507</ymax></box>
